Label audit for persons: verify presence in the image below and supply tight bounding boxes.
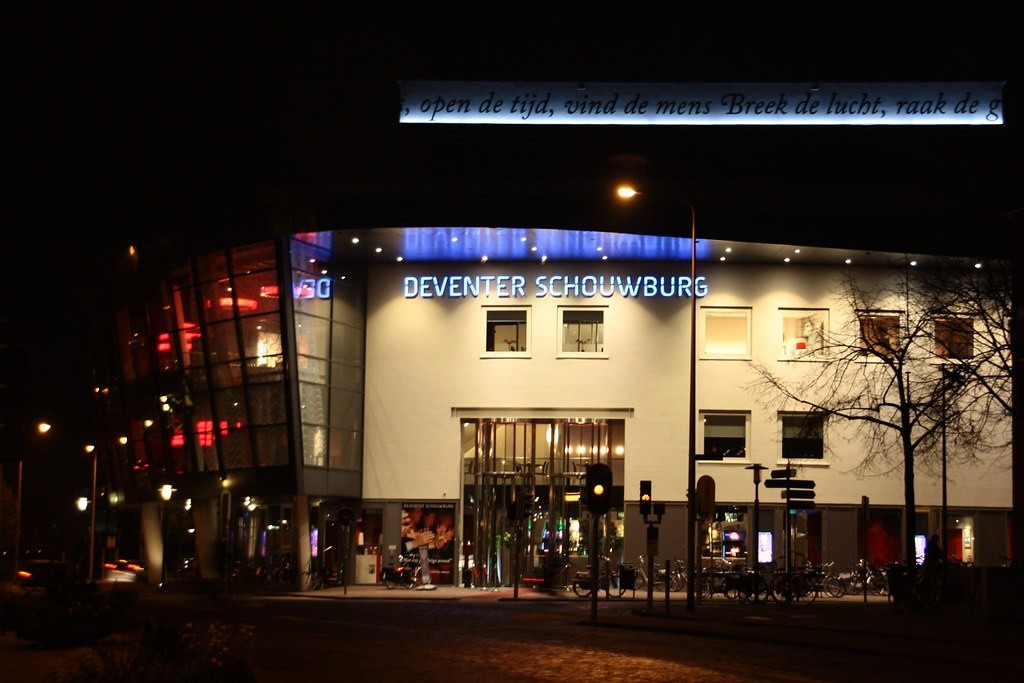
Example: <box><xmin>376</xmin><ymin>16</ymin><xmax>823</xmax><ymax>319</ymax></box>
<box><xmin>925</xmin><ymin>535</ymin><xmax>945</xmax><ymax>569</ymax></box>
<box><xmin>400</xmin><ymin>505</ymin><xmax>454</xmax><ymax>560</ymax></box>
<box><xmin>542</xmin><ymin>523</ymin><xmax>575</xmax><ymax>553</ymax></box>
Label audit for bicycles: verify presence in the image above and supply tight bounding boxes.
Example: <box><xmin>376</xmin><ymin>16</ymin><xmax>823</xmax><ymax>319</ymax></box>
<box><xmin>382</xmin><ymin>556</ymin><xmax>420</xmax><ymax>590</ymax></box>
<box><xmin>571</xmin><ymin>552</ymin><xmax>1007</xmax><ymax>601</ymax></box>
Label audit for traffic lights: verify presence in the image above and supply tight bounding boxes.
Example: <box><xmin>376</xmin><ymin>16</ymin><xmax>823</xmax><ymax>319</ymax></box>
<box><xmin>788</xmin><ymin>506</ymin><xmax>798</xmax><ymax>518</ymax></box>
<box><xmin>722</xmin><ymin>530</ymin><xmax>745</xmax><ymax>542</ymax></box>
<box><xmin>638</xmin><ymin>480</ymin><xmax>651</xmax><ymax>515</ymax></box>
<box><xmin>584</xmin><ymin>462</ymin><xmax>611</xmax><ymax>516</ymax></box>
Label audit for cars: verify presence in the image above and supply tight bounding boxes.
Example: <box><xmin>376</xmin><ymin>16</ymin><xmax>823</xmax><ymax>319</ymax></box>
<box><xmin>1</xmin><ymin>554</ymin><xmax>348</xmax><ymax>648</ymax></box>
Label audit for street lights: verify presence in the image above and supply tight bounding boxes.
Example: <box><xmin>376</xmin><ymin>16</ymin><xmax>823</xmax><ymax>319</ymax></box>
<box><xmin>82</xmin><ymin>444</ymin><xmax>99</xmax><ymax>578</ymax></box>
<box><xmin>940</xmin><ymin>371</ymin><xmax>963</xmax><ymax>562</ymax></box>
<box><xmin>610</xmin><ymin>177</ymin><xmax>698</xmax><ymax>612</ymax></box>
<box><xmin>746</xmin><ymin>464</ymin><xmax>770</xmax><ymax>601</ymax></box>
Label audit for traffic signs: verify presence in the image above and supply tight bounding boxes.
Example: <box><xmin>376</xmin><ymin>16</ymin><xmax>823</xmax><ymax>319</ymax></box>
<box><xmin>771</xmin><ymin>469</ymin><xmax>797</xmax><ymax>479</ymax></box>
<box><xmin>780</xmin><ymin>490</ymin><xmax>817</xmax><ymax>500</ymax></box>
<box><xmin>764</xmin><ymin>478</ymin><xmax>816</xmax><ymax>490</ymax></box>
<box><xmin>786</xmin><ymin>500</ymin><xmax>816</xmax><ymax>510</ymax></box>
